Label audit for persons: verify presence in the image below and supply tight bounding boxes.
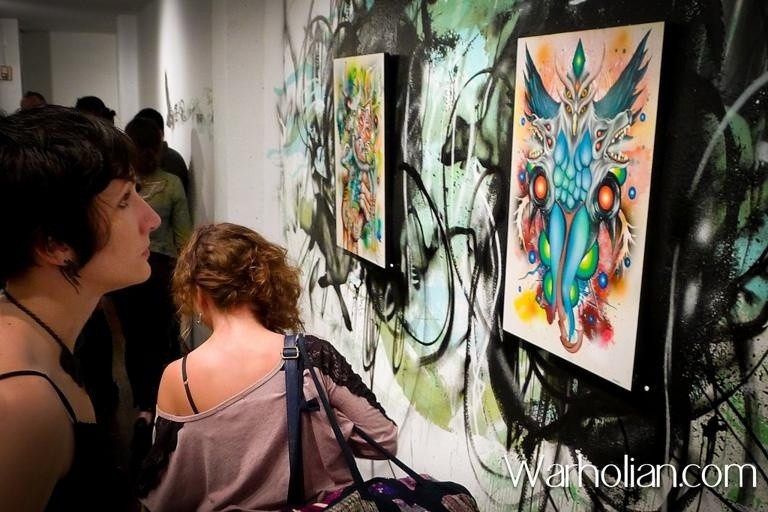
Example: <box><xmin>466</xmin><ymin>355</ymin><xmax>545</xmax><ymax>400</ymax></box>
<box><xmin>0</xmin><ymin>103</ymin><xmax>164</xmax><ymax>512</ymax></box>
<box><xmin>136</xmin><ymin>107</ymin><xmax>189</xmax><ymax>196</ymax></box>
<box><xmin>111</xmin><ymin>116</ymin><xmax>193</xmax><ymax>459</ymax></box>
<box><xmin>134</xmin><ymin>220</ymin><xmax>399</xmax><ymax>511</ymax></box>
<box><xmin>74</xmin><ymin>96</ymin><xmax>116</xmax><ymax>125</ymax></box>
<box><xmin>20</xmin><ymin>91</ymin><xmax>48</xmax><ymax>112</ymax></box>
<box><xmin>75</xmin><ymin>293</ymin><xmax>119</xmax><ymax>407</ymax></box>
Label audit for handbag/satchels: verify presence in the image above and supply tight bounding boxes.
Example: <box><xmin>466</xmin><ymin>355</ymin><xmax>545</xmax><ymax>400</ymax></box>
<box><xmin>292</xmin><ymin>473</ymin><xmax>479</xmax><ymax>512</ymax></box>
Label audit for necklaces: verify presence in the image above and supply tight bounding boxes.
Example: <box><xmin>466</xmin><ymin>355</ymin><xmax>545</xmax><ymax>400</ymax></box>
<box><xmin>0</xmin><ymin>286</ymin><xmax>86</xmax><ymax>390</ymax></box>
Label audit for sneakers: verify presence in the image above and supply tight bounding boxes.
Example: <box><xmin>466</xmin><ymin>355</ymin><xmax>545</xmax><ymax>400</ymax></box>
<box><xmin>128</xmin><ymin>418</ymin><xmax>148</xmax><ymax>455</ymax></box>
